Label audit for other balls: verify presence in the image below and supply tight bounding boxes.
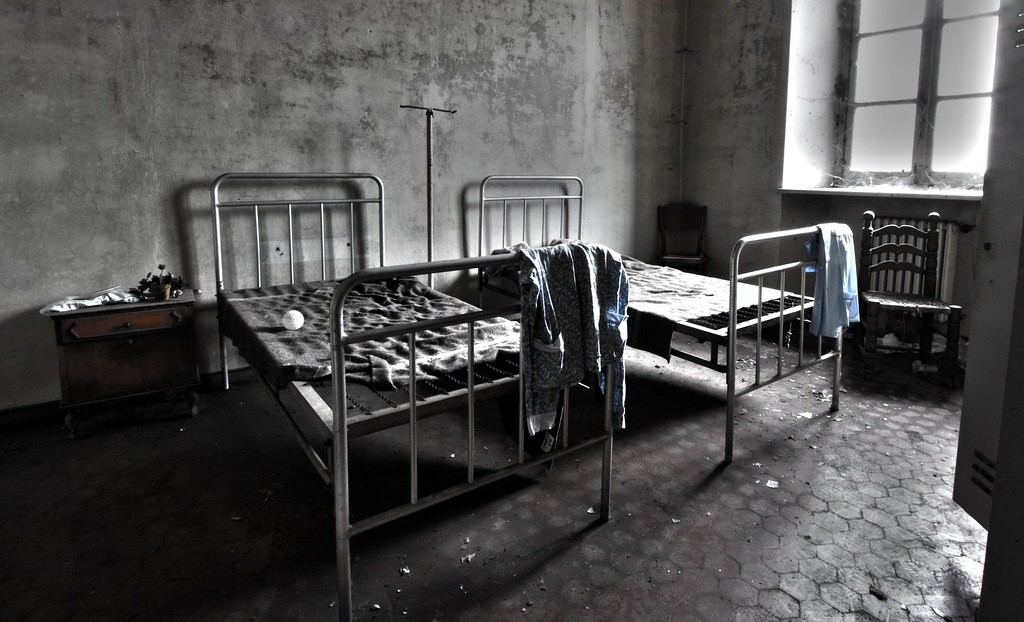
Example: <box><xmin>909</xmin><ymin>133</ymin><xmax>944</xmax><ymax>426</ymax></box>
<box><xmin>282</xmin><ymin>309</ymin><xmax>304</xmax><ymax>331</ymax></box>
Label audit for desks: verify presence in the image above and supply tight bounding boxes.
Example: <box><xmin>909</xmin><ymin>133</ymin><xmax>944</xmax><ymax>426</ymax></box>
<box><xmin>49</xmin><ymin>288</ymin><xmax>202</xmax><ymax>438</ymax></box>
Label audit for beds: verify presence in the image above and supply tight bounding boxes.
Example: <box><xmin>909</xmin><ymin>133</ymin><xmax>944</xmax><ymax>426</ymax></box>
<box><xmin>211</xmin><ymin>172</ymin><xmax>615</xmax><ymax>622</ymax></box>
<box><xmin>477</xmin><ymin>172</ymin><xmax>844</xmax><ymax>464</ymax></box>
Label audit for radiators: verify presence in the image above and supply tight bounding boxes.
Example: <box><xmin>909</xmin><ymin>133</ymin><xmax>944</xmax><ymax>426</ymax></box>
<box><xmin>871</xmin><ymin>214</ymin><xmax>968</xmax><ymax>322</ymax></box>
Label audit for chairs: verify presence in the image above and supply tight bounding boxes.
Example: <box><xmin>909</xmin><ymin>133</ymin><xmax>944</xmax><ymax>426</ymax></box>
<box><xmin>851</xmin><ymin>211</ymin><xmax>963</xmax><ymax>392</ymax></box>
<box><xmin>650</xmin><ymin>200</ymin><xmax>711</xmax><ymax>278</ymax></box>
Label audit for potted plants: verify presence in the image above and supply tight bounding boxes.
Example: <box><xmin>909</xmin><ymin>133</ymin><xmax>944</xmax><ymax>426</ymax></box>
<box><xmin>128</xmin><ymin>263</ymin><xmax>185</xmax><ymax>302</ymax></box>
